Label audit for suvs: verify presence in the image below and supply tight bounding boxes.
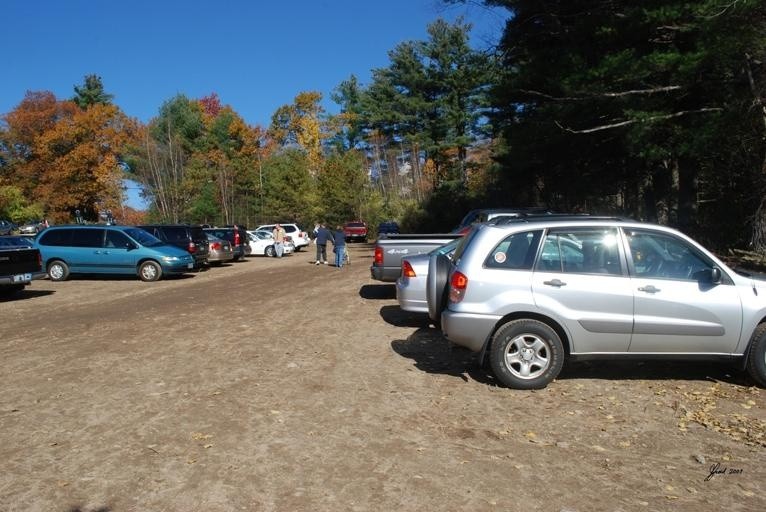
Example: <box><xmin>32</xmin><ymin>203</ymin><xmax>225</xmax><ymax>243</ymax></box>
<box><xmin>424</xmin><ymin>209</ymin><xmax>766</xmax><ymax>391</ymax></box>
<box><xmin>199</xmin><ymin>224</ymin><xmax>251</xmax><ymax>262</ymax></box>
<box><xmin>449</xmin><ymin>210</ymin><xmax>482</xmax><ymax>234</ymax></box>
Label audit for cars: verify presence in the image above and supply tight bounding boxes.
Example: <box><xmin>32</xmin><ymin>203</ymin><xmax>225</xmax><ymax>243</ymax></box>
<box><xmin>255</xmin><ymin>223</ymin><xmax>311</xmax><ymax>252</ymax></box>
<box><xmin>376</xmin><ymin>221</ymin><xmax>401</xmax><ymax>235</ymax></box>
<box><xmin>18</xmin><ymin>221</ymin><xmax>45</xmax><ymax>234</ymax></box>
<box><xmin>255</xmin><ymin>229</ymin><xmax>294</xmax><ymax>245</ymax></box>
<box><xmin>395</xmin><ymin>233</ymin><xmax>583</xmax><ymax>313</ymax></box>
<box><xmin>0</xmin><ymin>236</ymin><xmax>35</xmax><ymax>250</ymax></box>
<box><xmin>202</xmin><ymin>231</ymin><xmax>233</xmax><ymax>267</ymax></box>
<box><xmin>243</xmin><ymin>231</ymin><xmax>294</xmax><ymax>257</ymax></box>
<box><xmin>0</xmin><ymin>220</ymin><xmax>14</xmax><ymax>236</ymax></box>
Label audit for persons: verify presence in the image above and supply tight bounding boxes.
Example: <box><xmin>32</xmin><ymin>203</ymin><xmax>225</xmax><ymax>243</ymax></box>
<box><xmin>272</xmin><ymin>223</ymin><xmax>286</xmax><ymax>259</ymax></box>
<box><xmin>233</xmin><ymin>220</ymin><xmax>246</xmax><ymax>260</ymax></box>
<box><xmin>312</xmin><ymin>222</ymin><xmax>351</xmax><ymax>267</ymax></box>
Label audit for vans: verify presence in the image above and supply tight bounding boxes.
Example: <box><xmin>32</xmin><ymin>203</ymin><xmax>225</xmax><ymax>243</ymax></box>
<box><xmin>32</xmin><ymin>224</ymin><xmax>195</xmax><ymax>282</ymax></box>
<box><xmin>130</xmin><ymin>224</ymin><xmax>210</xmax><ymax>277</ymax></box>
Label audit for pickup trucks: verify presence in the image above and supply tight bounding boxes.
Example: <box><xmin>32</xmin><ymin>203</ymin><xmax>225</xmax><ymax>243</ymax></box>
<box><xmin>343</xmin><ymin>222</ymin><xmax>368</xmax><ymax>243</ymax></box>
<box><xmin>370</xmin><ymin>210</ymin><xmax>560</xmax><ymax>283</ymax></box>
<box><xmin>0</xmin><ymin>245</ymin><xmax>47</xmax><ymax>297</ymax></box>
